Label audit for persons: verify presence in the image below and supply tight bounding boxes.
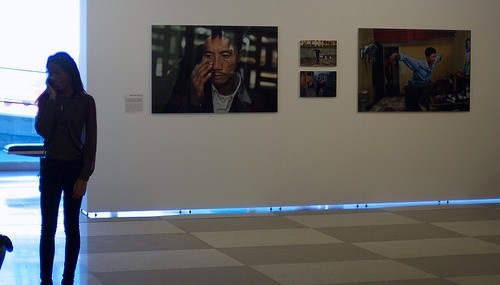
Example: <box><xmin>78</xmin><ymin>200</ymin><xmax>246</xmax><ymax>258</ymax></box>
<box><xmin>464</xmin><ymin>38</ymin><xmax>471</xmax><ymax>92</ymax></box>
<box><xmin>302</xmin><ymin>81</ymin><xmax>318</xmax><ymax>97</ymax></box>
<box><xmin>35</xmin><ymin>51</ymin><xmax>97</xmax><ymax>285</ymax></box>
<box><xmin>390</xmin><ymin>46</ymin><xmax>444</xmax><ymax>111</ymax></box>
<box><xmin>313</xmin><ymin>47</ymin><xmax>321</xmax><ymax>61</ymax></box>
<box><xmin>173</xmin><ymin>26</ymin><xmax>274</xmax><ymax>113</ymax></box>
